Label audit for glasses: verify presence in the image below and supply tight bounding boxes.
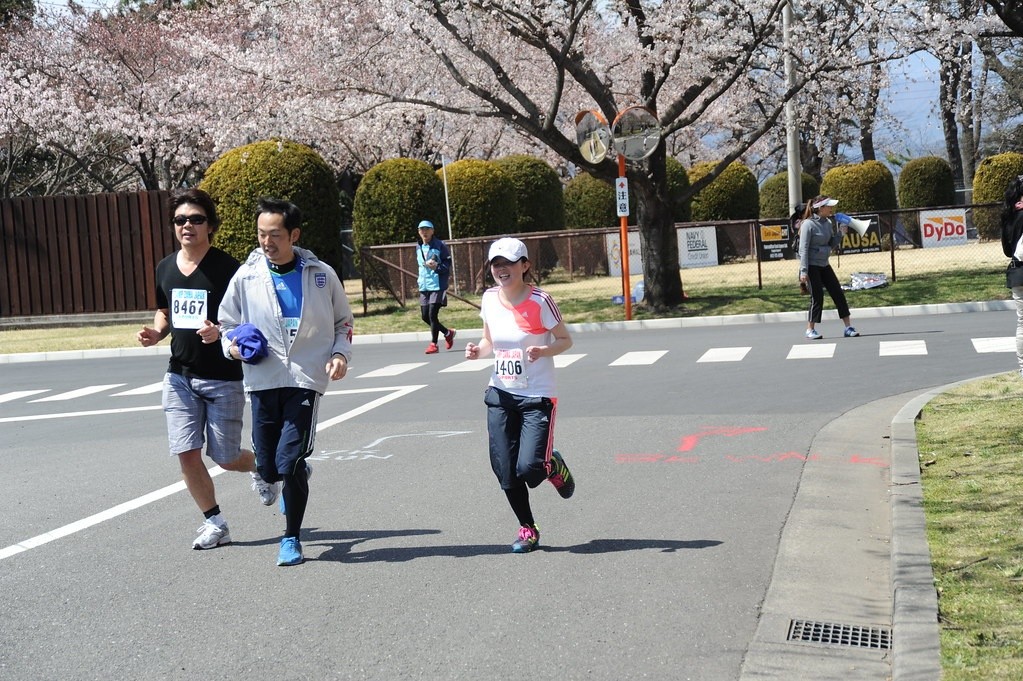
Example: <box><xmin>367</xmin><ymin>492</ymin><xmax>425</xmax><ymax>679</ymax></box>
<box><xmin>172</xmin><ymin>214</ymin><xmax>209</xmax><ymax>226</ymax></box>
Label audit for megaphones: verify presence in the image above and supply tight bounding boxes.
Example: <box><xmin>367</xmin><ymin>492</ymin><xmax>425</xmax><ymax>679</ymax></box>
<box><xmin>833</xmin><ymin>213</ymin><xmax>871</xmax><ymax>239</ymax></box>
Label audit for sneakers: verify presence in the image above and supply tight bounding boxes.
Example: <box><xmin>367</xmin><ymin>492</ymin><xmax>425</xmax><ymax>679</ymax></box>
<box><xmin>843</xmin><ymin>326</ymin><xmax>859</xmax><ymax>337</ymax></box>
<box><xmin>806</xmin><ymin>329</ymin><xmax>822</xmax><ymax>339</ymax></box>
<box><xmin>425</xmin><ymin>342</ymin><xmax>439</xmax><ymax>354</ymax></box>
<box><xmin>548</xmin><ymin>450</ymin><xmax>575</xmax><ymax>499</ymax></box>
<box><xmin>444</xmin><ymin>328</ymin><xmax>456</xmax><ymax>349</ymax></box>
<box><xmin>249</xmin><ymin>450</ymin><xmax>277</xmax><ymax>506</ymax></box>
<box><xmin>192</xmin><ymin>520</ymin><xmax>231</xmax><ymax>549</ymax></box>
<box><xmin>280</xmin><ymin>460</ymin><xmax>313</xmax><ymax>515</ymax></box>
<box><xmin>277</xmin><ymin>534</ymin><xmax>304</xmax><ymax>565</ymax></box>
<box><xmin>511</xmin><ymin>524</ymin><xmax>539</xmax><ymax>553</ymax></box>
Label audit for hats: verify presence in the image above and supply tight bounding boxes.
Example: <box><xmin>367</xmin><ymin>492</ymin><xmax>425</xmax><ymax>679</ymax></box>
<box><xmin>488</xmin><ymin>237</ymin><xmax>528</xmax><ymax>264</ymax></box>
<box><xmin>813</xmin><ymin>198</ymin><xmax>839</xmax><ymax>209</ymax></box>
<box><xmin>418</xmin><ymin>220</ymin><xmax>433</xmax><ymax>229</ymax></box>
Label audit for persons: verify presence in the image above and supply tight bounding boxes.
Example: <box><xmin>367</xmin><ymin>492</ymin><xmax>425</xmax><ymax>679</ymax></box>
<box><xmin>789</xmin><ymin>205</ymin><xmax>802</xmax><ymax>260</ymax></box>
<box><xmin>799</xmin><ymin>194</ymin><xmax>860</xmax><ymax>339</ymax></box>
<box><xmin>620</xmin><ymin>125</ymin><xmax>651</xmax><ymax>155</ymax></box>
<box><xmin>416</xmin><ymin>220</ymin><xmax>457</xmax><ymax>354</ymax></box>
<box><xmin>1000</xmin><ymin>176</ymin><xmax>1023</xmax><ymax>371</ymax></box>
<box><xmin>466</xmin><ymin>238</ymin><xmax>576</xmax><ymax>553</ymax></box>
<box><xmin>137</xmin><ymin>190</ymin><xmax>354</xmax><ymax>567</ymax></box>
